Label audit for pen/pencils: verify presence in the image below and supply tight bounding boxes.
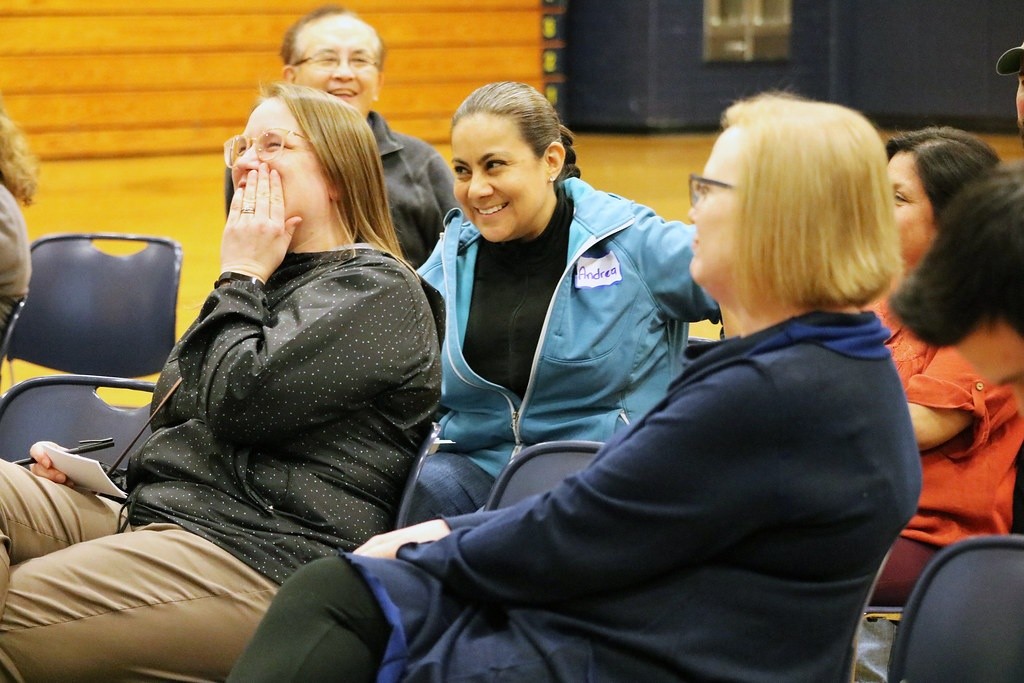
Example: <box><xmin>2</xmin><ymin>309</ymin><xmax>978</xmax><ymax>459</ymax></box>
<box><xmin>11</xmin><ymin>437</ymin><xmax>115</xmax><ymax>467</ymax></box>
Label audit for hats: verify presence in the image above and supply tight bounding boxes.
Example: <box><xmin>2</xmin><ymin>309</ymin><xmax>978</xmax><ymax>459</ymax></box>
<box><xmin>996</xmin><ymin>40</ymin><xmax>1024</xmax><ymax>77</ymax></box>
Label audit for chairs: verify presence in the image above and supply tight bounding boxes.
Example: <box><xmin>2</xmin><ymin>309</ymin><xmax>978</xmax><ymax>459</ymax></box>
<box><xmin>887</xmin><ymin>535</ymin><xmax>1024</xmax><ymax>683</ymax></box>
<box><xmin>0</xmin><ymin>233</ymin><xmax>183</xmax><ymax>387</ymax></box>
<box><xmin>481</xmin><ymin>439</ymin><xmax>604</xmax><ymax>511</ymax></box>
<box><xmin>0</xmin><ymin>373</ymin><xmax>156</xmax><ymax>469</ymax></box>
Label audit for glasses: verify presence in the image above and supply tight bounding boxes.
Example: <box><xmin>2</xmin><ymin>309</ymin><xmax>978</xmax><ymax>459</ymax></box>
<box><xmin>222</xmin><ymin>128</ymin><xmax>311</xmax><ymax>169</ymax></box>
<box><xmin>687</xmin><ymin>170</ymin><xmax>737</xmax><ymax>207</ymax></box>
<box><xmin>291</xmin><ymin>47</ymin><xmax>381</xmax><ymax>70</ymax></box>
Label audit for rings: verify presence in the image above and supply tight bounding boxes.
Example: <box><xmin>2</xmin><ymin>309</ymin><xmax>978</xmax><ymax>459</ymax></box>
<box><xmin>242</xmin><ymin>208</ymin><xmax>254</xmax><ymax>213</ymax></box>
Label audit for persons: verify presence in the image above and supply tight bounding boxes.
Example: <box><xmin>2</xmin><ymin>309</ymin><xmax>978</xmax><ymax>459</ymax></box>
<box><xmin>0</xmin><ymin>86</ymin><xmax>443</xmax><ymax>683</ymax></box>
<box><xmin>0</xmin><ymin>108</ymin><xmax>39</xmax><ymax>342</ymax></box>
<box><xmin>871</xmin><ymin>40</ymin><xmax>1024</xmax><ymax>607</ymax></box>
<box><xmin>410</xmin><ymin>81</ymin><xmax>721</xmax><ymax>527</ymax></box>
<box><xmin>227</xmin><ymin>9</ymin><xmax>460</xmax><ymax>269</ymax></box>
<box><xmin>226</xmin><ymin>95</ymin><xmax>923</xmax><ymax>683</ymax></box>
